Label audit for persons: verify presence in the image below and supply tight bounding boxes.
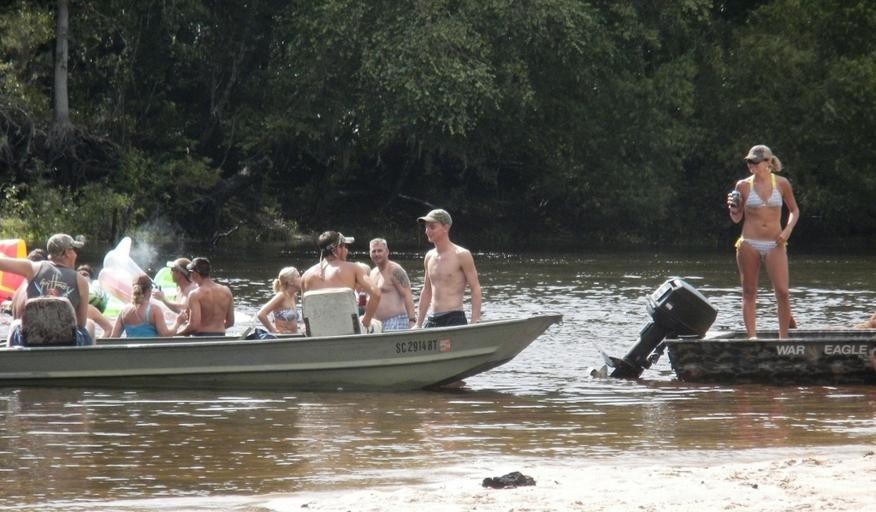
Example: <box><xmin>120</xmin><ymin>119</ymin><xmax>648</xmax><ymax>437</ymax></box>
<box><xmin>364</xmin><ymin>238</ymin><xmax>417</xmax><ymax>331</ymax></box>
<box><xmin>300</xmin><ymin>231</ymin><xmax>382</xmax><ymax>334</ymax></box>
<box><xmin>0</xmin><ymin>234</ymin><xmax>95</xmax><ymax>348</ymax></box>
<box><xmin>257</xmin><ymin>267</ymin><xmax>300</xmax><ymax>334</ymax></box>
<box><xmin>345</xmin><ymin>243</ymin><xmax>370</xmax><ymax>317</ymax></box>
<box><xmin>76</xmin><ymin>265</ymin><xmax>107</xmax><ymax>314</ymax></box>
<box><xmin>78</xmin><ymin>271</ymin><xmax>113</xmax><ymax>337</ymax></box>
<box><xmin>410</xmin><ymin>209</ymin><xmax>481</xmax><ymax>329</ymax></box>
<box><xmin>151</xmin><ymin>258</ymin><xmax>199</xmax><ymax>321</ymax></box>
<box><xmin>727</xmin><ymin>145</ymin><xmax>799</xmax><ymax>340</ymax></box>
<box><xmin>11</xmin><ymin>249</ymin><xmax>48</xmax><ymax>320</ymax></box>
<box><xmin>109</xmin><ymin>275</ymin><xmax>188</xmax><ymax>338</ymax></box>
<box><xmin>176</xmin><ymin>257</ymin><xmax>234</xmax><ymax>336</ymax></box>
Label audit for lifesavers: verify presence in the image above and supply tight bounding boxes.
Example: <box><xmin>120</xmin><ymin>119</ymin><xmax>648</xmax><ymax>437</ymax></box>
<box><xmin>98</xmin><ymin>236</ymin><xmax>159</xmax><ymax>303</ymax></box>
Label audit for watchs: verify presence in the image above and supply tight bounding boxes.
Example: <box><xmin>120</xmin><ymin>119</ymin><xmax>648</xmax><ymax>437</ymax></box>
<box><xmin>409</xmin><ymin>317</ymin><xmax>417</xmax><ymax>323</ymax></box>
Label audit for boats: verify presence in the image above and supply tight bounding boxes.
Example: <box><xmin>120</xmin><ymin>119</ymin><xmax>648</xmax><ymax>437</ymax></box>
<box><xmin>0</xmin><ymin>313</ymin><xmax>564</xmax><ymax>391</ymax></box>
<box><xmin>590</xmin><ymin>279</ymin><xmax>876</xmax><ymax>385</ymax></box>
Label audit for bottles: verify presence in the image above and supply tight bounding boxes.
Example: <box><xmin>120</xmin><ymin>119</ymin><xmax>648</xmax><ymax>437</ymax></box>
<box><xmin>728</xmin><ymin>189</ymin><xmax>742</xmax><ymax>208</ymax></box>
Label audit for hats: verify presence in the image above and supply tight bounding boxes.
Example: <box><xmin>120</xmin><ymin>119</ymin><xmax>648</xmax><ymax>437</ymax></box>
<box><xmin>319</xmin><ymin>232</ymin><xmax>354</xmax><ymax>249</ymax></box>
<box><xmin>186</xmin><ymin>263</ymin><xmax>212</xmax><ymax>273</ymax></box>
<box><xmin>417</xmin><ymin>208</ymin><xmax>453</xmax><ymax>225</ymax></box>
<box><xmin>47</xmin><ymin>234</ymin><xmax>84</xmax><ymax>255</ymax></box>
<box><xmin>743</xmin><ymin>144</ymin><xmax>772</xmax><ymax>163</ymax></box>
<box><xmin>167</xmin><ymin>259</ymin><xmax>187</xmax><ymax>276</ymax></box>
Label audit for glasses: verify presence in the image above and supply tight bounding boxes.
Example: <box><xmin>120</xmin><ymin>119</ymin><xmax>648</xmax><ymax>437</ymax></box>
<box><xmin>746</xmin><ymin>158</ymin><xmax>760</xmax><ymax>164</ymax></box>
<box><xmin>81</xmin><ymin>273</ymin><xmax>90</xmax><ymax>277</ymax></box>
